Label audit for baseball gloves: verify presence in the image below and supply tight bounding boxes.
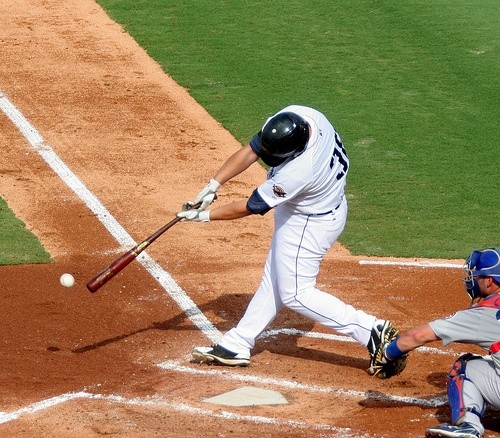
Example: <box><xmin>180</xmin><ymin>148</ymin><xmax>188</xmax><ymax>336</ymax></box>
<box><xmin>370</xmin><ymin>321</ymin><xmax>408</xmax><ymax>380</ymax></box>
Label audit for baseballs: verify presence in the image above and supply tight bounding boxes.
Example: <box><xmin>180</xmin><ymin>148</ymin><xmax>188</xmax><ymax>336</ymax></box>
<box><xmin>59</xmin><ymin>272</ymin><xmax>75</xmax><ymax>288</ymax></box>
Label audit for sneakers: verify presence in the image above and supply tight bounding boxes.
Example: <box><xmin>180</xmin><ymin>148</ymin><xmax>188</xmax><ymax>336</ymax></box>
<box><xmin>367</xmin><ymin>319</ymin><xmax>392</xmax><ymax>375</ymax></box>
<box><xmin>191</xmin><ymin>344</ymin><xmax>251</xmax><ymax>367</ymax></box>
<box><xmin>425</xmin><ymin>422</ymin><xmax>481</xmax><ymax>438</ymax></box>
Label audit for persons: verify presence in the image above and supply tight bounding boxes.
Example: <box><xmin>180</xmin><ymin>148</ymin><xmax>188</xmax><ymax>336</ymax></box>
<box><xmin>382</xmin><ymin>248</ymin><xmax>500</xmax><ymax>438</ymax></box>
<box><xmin>176</xmin><ymin>105</ymin><xmax>408</xmax><ymax>377</ymax></box>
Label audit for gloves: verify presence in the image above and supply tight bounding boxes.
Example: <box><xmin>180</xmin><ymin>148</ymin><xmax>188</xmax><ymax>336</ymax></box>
<box><xmin>194</xmin><ymin>178</ymin><xmax>222</xmax><ymax>210</ymax></box>
<box><xmin>176</xmin><ymin>201</ymin><xmax>211</xmax><ymax>222</ymax></box>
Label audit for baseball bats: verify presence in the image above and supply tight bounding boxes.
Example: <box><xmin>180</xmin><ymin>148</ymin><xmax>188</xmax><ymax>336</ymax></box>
<box><xmin>86</xmin><ymin>196</ymin><xmax>214</xmax><ymax>294</ymax></box>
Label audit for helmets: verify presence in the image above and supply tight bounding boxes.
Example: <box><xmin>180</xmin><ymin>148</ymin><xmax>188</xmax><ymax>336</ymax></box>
<box><xmin>259</xmin><ymin>112</ymin><xmax>309</xmax><ymax>167</ymax></box>
<box><xmin>463</xmin><ymin>248</ymin><xmax>500</xmax><ymax>300</ymax></box>
